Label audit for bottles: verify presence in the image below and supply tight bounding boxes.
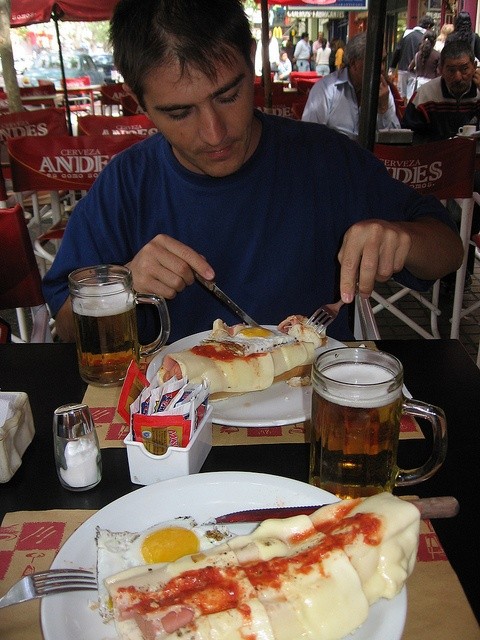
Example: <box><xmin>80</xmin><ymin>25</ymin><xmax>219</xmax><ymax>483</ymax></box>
<box><xmin>53</xmin><ymin>403</ymin><xmax>103</xmax><ymax>492</ymax></box>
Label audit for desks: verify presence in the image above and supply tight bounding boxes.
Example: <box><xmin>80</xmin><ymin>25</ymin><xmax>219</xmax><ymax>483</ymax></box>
<box><xmin>0</xmin><ymin>78</ymin><xmax>56</xmax><ymax>111</ymax></box>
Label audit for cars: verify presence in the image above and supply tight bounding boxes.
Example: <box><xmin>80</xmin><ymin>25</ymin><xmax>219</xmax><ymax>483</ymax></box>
<box><xmin>29</xmin><ymin>49</ymin><xmax>105</xmax><ymax>104</ymax></box>
<box><xmin>92</xmin><ymin>54</ymin><xmax>120</xmax><ymax>84</ymax></box>
<box><xmin>0</xmin><ymin>57</ymin><xmax>28</xmax><ymax>88</ymax></box>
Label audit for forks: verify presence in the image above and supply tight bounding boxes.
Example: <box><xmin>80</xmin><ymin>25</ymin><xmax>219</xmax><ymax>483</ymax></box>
<box><xmin>306</xmin><ymin>284</ymin><xmax>359</xmax><ymax>334</ymax></box>
<box><xmin>0</xmin><ymin>568</ymin><xmax>98</xmax><ymax>608</ymax></box>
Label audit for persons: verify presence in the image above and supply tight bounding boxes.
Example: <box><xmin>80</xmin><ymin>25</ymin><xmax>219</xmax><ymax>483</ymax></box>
<box><xmin>388</xmin><ymin>14</ymin><xmax>434</xmax><ymax>105</ymax></box>
<box><xmin>444</xmin><ymin>11</ymin><xmax>480</xmax><ymax>62</ymax></box>
<box><xmin>312</xmin><ymin>32</ymin><xmax>329</xmax><ymax>69</ymax></box>
<box><xmin>409</xmin><ymin>33</ymin><xmax>441</xmax><ymax>78</ymax></box>
<box><xmin>403</xmin><ymin>41</ymin><xmax>480</xmax><ymax>140</ymax></box>
<box><xmin>287</xmin><ymin>34</ymin><xmax>294</xmax><ymax>48</ymax></box>
<box><xmin>294</xmin><ymin>33</ymin><xmax>312</xmax><ymax>72</ymax></box>
<box><xmin>301</xmin><ymin>33</ymin><xmax>401</xmax><ymax>143</ymax></box>
<box><xmin>276</xmin><ymin>51</ymin><xmax>292</xmax><ymax>80</ymax></box>
<box><xmin>42</xmin><ymin>0</ymin><xmax>464</xmax><ymax>341</ymax></box>
<box><xmin>334</xmin><ymin>39</ymin><xmax>345</xmax><ymax>71</ymax></box>
<box><xmin>254</xmin><ymin>28</ymin><xmax>279</xmax><ymax>73</ymax></box>
<box><xmin>315</xmin><ymin>38</ymin><xmax>332</xmax><ymax>76</ymax></box>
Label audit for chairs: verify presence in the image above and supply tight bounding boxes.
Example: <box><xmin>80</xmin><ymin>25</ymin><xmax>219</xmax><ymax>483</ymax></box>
<box><xmin>98</xmin><ymin>82</ymin><xmax>131</xmax><ymax>117</ymax></box>
<box><xmin>121</xmin><ymin>94</ymin><xmax>138</xmax><ymax>117</ymax></box>
<box><xmin>349</xmin><ymin>135</ymin><xmax>475</xmax><ymax>339</ymax></box>
<box><xmin>60</xmin><ymin>75</ymin><xmax>97</xmax><ymax>129</ymax></box>
<box><xmin>0</xmin><ymin>203</ymin><xmax>52</xmax><ymax>344</ymax></box>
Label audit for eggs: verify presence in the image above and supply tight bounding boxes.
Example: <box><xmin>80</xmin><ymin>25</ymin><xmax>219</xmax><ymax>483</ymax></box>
<box><xmin>122</xmin><ymin>519</ymin><xmax>213</xmax><ymax>567</ymax></box>
<box><xmin>235</xmin><ymin>324</ymin><xmax>293</xmax><ymax>346</ymax></box>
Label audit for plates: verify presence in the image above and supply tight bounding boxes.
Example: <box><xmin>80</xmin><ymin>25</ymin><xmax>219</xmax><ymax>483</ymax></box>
<box><xmin>146</xmin><ymin>324</ymin><xmax>348</xmax><ymax>428</ymax></box>
<box><xmin>456</xmin><ymin>131</ymin><xmax>480</xmax><ymax>137</ymax></box>
<box><xmin>42</xmin><ymin>471</ymin><xmax>408</xmax><ymax>635</ymax></box>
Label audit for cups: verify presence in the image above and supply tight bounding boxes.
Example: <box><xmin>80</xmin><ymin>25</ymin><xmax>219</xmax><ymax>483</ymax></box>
<box><xmin>308</xmin><ymin>346</ymin><xmax>448</xmax><ymax>501</ymax></box>
<box><xmin>68</xmin><ymin>263</ymin><xmax>171</xmax><ymax>388</ymax></box>
<box><xmin>458</xmin><ymin>125</ymin><xmax>477</xmax><ymax>135</ymax></box>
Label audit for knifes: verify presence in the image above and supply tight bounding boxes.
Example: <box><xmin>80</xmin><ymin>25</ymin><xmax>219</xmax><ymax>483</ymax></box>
<box><xmin>201</xmin><ymin>496</ymin><xmax>460</xmax><ymax>523</ymax></box>
<box><xmin>196</xmin><ymin>273</ymin><xmax>259</xmax><ymax>328</ymax></box>
